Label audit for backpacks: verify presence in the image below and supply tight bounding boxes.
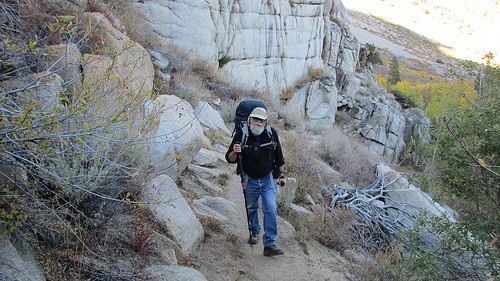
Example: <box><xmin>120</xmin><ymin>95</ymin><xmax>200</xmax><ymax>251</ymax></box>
<box><xmin>230</xmin><ymin>99</ymin><xmax>277</xmax><ymax>150</ymax></box>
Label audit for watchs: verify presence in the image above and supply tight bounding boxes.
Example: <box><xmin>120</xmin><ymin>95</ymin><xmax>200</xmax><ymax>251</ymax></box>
<box><xmin>279</xmin><ymin>175</ymin><xmax>285</xmax><ymax>180</ymax></box>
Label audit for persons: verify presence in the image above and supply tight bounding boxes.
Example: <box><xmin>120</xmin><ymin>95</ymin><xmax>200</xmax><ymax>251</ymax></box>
<box><xmin>225</xmin><ymin>107</ymin><xmax>286</xmax><ymax>257</ymax></box>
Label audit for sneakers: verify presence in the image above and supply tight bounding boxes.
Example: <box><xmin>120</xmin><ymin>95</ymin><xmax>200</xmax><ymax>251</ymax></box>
<box><xmin>264</xmin><ymin>244</ymin><xmax>284</xmax><ymax>256</ymax></box>
<box><xmin>248</xmin><ymin>232</ymin><xmax>259</xmax><ymax>244</ymax></box>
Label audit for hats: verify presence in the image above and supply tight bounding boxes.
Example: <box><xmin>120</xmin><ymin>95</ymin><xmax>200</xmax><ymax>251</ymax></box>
<box><xmin>250</xmin><ymin>107</ymin><xmax>269</xmax><ymax>121</ymax></box>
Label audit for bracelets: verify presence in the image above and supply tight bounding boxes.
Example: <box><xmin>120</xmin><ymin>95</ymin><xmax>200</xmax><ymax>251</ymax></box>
<box><xmin>280</xmin><ymin>171</ymin><xmax>285</xmax><ymax>174</ymax></box>
<box><xmin>233</xmin><ymin>150</ymin><xmax>238</xmax><ymax>154</ymax></box>
